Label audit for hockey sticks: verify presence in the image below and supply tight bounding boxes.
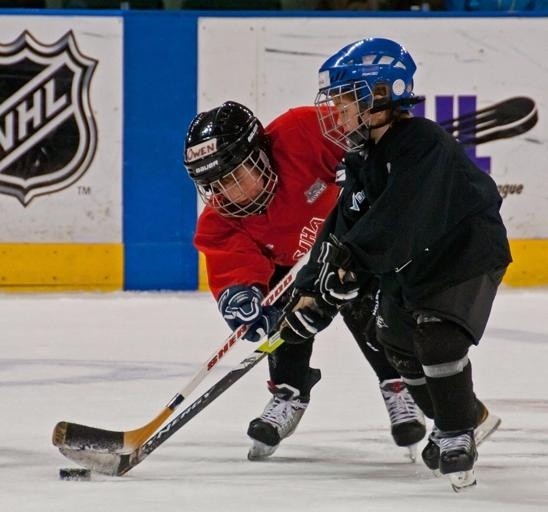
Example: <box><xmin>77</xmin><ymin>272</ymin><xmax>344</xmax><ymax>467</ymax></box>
<box><xmin>59</xmin><ymin>271</ymin><xmax>356</xmax><ymax>477</ymax></box>
<box><xmin>51</xmin><ymin>242</ymin><xmax>311</xmax><ymax>454</ymax></box>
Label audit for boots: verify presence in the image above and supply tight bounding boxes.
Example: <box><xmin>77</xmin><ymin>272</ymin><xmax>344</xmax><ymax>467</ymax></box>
<box><xmin>421</xmin><ymin>399</ymin><xmax>501</xmax><ymax>474</ymax></box>
<box><xmin>246</xmin><ymin>368</ymin><xmax>321</xmax><ymax>447</ymax></box>
<box><xmin>378</xmin><ymin>379</ymin><xmax>426</xmax><ymax>447</ymax></box>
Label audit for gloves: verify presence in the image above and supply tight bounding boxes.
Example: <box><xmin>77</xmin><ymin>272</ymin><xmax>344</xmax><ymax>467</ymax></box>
<box><xmin>278</xmin><ymin>301</ymin><xmax>334</xmax><ymax>345</ymax></box>
<box><xmin>216</xmin><ymin>284</ymin><xmax>281</xmax><ymax>343</ymax></box>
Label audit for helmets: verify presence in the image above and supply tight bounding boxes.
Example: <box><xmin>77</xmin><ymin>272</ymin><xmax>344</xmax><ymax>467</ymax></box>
<box><xmin>181</xmin><ymin>99</ymin><xmax>265</xmax><ymax>187</ymax></box>
<box><xmin>317</xmin><ymin>37</ymin><xmax>418</xmax><ymax>110</ymax></box>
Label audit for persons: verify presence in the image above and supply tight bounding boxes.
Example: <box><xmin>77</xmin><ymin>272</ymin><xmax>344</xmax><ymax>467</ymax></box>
<box><xmin>281</xmin><ymin>38</ymin><xmax>513</xmax><ymax>474</ymax></box>
<box><xmin>183</xmin><ymin>101</ymin><xmax>427</xmax><ymax>446</ymax></box>
